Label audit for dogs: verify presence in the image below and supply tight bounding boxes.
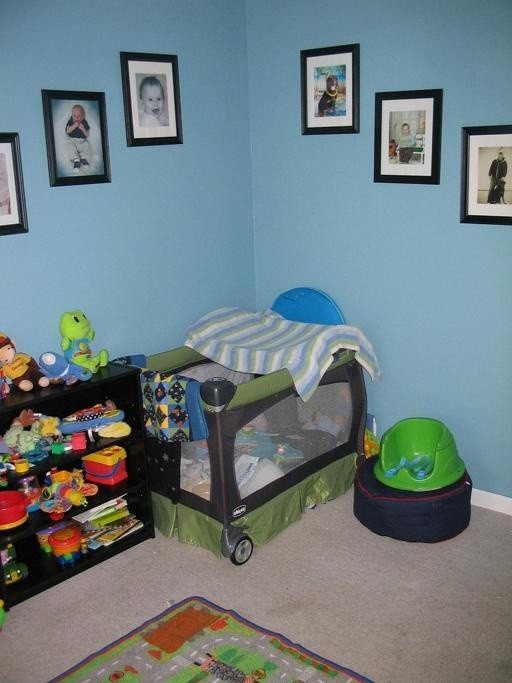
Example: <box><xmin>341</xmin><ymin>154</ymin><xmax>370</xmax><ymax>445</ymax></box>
<box><xmin>488</xmin><ymin>179</ymin><xmax>507</xmax><ymax>204</ymax></box>
<box><xmin>317</xmin><ymin>74</ymin><xmax>339</xmax><ymax>116</ymax></box>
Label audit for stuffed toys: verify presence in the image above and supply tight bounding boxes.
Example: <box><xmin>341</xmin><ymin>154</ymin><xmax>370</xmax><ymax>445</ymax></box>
<box><xmin>0</xmin><ymin>309</ymin><xmax>131</xmax><ymax>632</ymax></box>
<box><xmin>2</xmin><ymin>309</ymin><xmax>109</xmax><ymax>394</ymax></box>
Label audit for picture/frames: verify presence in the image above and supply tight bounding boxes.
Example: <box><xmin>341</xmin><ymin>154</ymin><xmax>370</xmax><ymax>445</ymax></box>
<box><xmin>0</xmin><ymin>131</ymin><xmax>29</xmax><ymax>237</ymax></box>
<box><xmin>40</xmin><ymin>88</ymin><xmax>111</xmax><ymax>187</ymax></box>
<box><xmin>373</xmin><ymin>87</ymin><xmax>443</xmax><ymax>185</ymax></box>
<box><xmin>119</xmin><ymin>50</ymin><xmax>183</xmax><ymax>147</ymax></box>
<box><xmin>460</xmin><ymin>125</ymin><xmax>512</xmax><ymax>225</ymax></box>
<box><xmin>298</xmin><ymin>43</ymin><xmax>360</xmax><ymax>135</ymax></box>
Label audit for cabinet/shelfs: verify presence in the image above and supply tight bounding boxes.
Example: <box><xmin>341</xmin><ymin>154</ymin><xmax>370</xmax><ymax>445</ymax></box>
<box><xmin>0</xmin><ymin>361</ymin><xmax>156</xmax><ymax>621</ymax></box>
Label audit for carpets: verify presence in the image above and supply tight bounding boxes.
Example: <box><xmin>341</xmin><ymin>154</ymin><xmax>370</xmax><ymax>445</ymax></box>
<box><xmin>43</xmin><ymin>592</ymin><xmax>375</xmax><ymax>682</ymax></box>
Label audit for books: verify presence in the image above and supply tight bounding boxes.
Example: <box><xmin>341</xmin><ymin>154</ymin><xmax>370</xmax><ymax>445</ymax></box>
<box><xmin>71</xmin><ymin>493</ymin><xmax>143</xmax><ymax>550</ymax></box>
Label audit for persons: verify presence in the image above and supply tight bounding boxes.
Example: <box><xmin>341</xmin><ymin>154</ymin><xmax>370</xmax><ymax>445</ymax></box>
<box><xmin>65</xmin><ymin>105</ymin><xmax>90</xmax><ymax>173</ymax></box>
<box><xmin>398</xmin><ymin>123</ymin><xmax>417</xmax><ymax>163</ymax></box>
<box><xmin>487</xmin><ymin>152</ymin><xmax>507</xmax><ymax>203</ymax></box>
<box><xmin>138</xmin><ymin>76</ymin><xmax>168</xmax><ymax>127</ymax></box>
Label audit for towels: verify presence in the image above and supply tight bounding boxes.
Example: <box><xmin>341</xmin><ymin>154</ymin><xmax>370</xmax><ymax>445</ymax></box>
<box><xmin>184</xmin><ymin>301</ymin><xmax>382</xmax><ymax>401</ymax></box>
